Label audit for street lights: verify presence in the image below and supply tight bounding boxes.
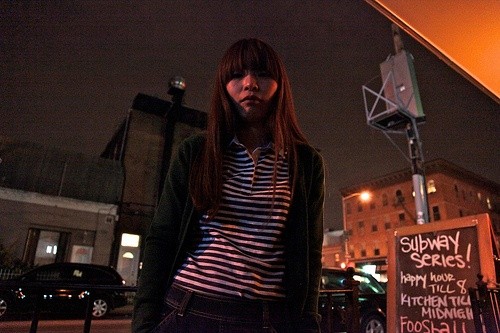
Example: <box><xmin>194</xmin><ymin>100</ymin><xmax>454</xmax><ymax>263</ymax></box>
<box><xmin>340</xmin><ymin>188</ymin><xmax>374</xmax><ymax>267</ymax></box>
<box><xmin>155</xmin><ymin>74</ymin><xmax>188</xmax><ymax>211</ymax></box>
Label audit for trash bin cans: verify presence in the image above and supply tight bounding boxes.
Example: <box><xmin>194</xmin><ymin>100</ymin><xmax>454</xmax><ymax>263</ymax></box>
<box><xmin>318</xmin><ymin>290</ymin><xmax>354</xmax><ymax>333</ymax></box>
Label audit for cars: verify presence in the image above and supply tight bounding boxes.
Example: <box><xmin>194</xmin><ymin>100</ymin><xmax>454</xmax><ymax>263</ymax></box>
<box><xmin>317</xmin><ymin>268</ymin><xmax>387</xmax><ymax>332</ymax></box>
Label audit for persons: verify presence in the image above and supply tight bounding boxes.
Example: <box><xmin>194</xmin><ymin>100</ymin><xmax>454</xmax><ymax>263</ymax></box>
<box><xmin>131</xmin><ymin>37</ymin><xmax>325</xmax><ymax>333</ymax></box>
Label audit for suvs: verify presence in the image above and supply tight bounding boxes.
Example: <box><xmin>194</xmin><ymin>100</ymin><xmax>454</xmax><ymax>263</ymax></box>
<box><xmin>0</xmin><ymin>263</ymin><xmax>127</xmax><ymax>319</ymax></box>
